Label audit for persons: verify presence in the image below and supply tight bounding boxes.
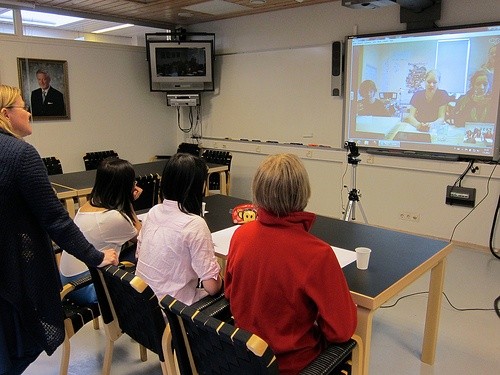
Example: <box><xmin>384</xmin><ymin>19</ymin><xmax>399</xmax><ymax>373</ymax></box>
<box><xmin>135</xmin><ymin>152</ymin><xmax>226</xmax><ymax>325</ymax></box>
<box><xmin>225</xmin><ymin>154</ymin><xmax>357</xmax><ymax>375</ymax></box>
<box><xmin>0</xmin><ymin>85</ymin><xmax>121</xmax><ymax>375</ymax></box>
<box><xmin>358</xmin><ymin>46</ymin><xmax>496</xmax><ymax>134</ymax></box>
<box><xmin>31</xmin><ymin>70</ymin><xmax>64</xmax><ymax>115</ymax></box>
<box><xmin>59</xmin><ymin>158</ymin><xmax>144</xmax><ymax>302</ymax></box>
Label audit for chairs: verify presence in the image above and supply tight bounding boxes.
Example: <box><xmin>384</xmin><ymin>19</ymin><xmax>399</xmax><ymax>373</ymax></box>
<box><xmin>96</xmin><ymin>263</ymin><xmax>223</xmax><ymax>375</ymax></box>
<box><xmin>160</xmin><ymin>294</ymin><xmax>364</xmax><ymax>375</ymax></box>
<box><xmin>83</xmin><ymin>142</ymin><xmax>233</xmax><ymax>214</ymax></box>
<box><xmin>43</xmin><ymin>157</ymin><xmax>63</xmax><ymax>175</ymax></box>
<box><xmin>52</xmin><ymin>243</ymin><xmax>135</xmax><ymax>375</ymax></box>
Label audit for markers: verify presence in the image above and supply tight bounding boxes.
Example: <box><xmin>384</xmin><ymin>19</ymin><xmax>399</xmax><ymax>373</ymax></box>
<box><xmin>290</xmin><ymin>142</ymin><xmax>304</xmax><ymax>145</ymax></box>
<box><xmin>308</xmin><ymin>143</ymin><xmax>317</xmax><ymax>146</ymax></box>
<box><xmin>265</xmin><ymin>141</ymin><xmax>278</xmax><ymax>143</ymax></box>
<box><xmin>319</xmin><ymin>144</ymin><xmax>331</xmax><ymax>147</ymax></box>
<box><xmin>239</xmin><ymin>138</ymin><xmax>248</xmax><ymax>141</ymax></box>
<box><xmin>252</xmin><ymin>139</ymin><xmax>260</xmax><ymax>142</ymax></box>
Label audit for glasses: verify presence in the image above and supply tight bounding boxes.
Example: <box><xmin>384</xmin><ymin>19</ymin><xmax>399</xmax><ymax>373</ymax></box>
<box><xmin>4</xmin><ymin>103</ymin><xmax>30</xmax><ymax>112</ymax></box>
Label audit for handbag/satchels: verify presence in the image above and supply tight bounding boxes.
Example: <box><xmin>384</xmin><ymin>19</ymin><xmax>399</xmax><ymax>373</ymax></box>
<box><xmin>231</xmin><ymin>204</ymin><xmax>259</xmax><ymax>224</ymax></box>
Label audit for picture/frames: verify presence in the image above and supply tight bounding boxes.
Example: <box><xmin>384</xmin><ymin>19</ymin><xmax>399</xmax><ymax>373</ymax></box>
<box><xmin>15</xmin><ymin>57</ymin><xmax>72</xmax><ymax>122</ymax></box>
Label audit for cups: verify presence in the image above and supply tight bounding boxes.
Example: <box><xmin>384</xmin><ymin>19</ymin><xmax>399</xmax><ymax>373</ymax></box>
<box><xmin>355</xmin><ymin>247</ymin><xmax>372</xmax><ymax>270</ymax></box>
<box><xmin>430</xmin><ymin>127</ymin><xmax>437</xmax><ymax>142</ymax></box>
<box><xmin>202</xmin><ymin>202</ymin><xmax>206</xmax><ymax>216</ymax></box>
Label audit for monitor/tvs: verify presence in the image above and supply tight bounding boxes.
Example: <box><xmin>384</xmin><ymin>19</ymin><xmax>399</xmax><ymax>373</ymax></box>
<box><xmin>145</xmin><ymin>42</ymin><xmax>216</xmax><ymax>91</ymax></box>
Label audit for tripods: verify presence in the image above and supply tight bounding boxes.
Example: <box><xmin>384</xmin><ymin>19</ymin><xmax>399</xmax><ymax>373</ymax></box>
<box><xmin>343</xmin><ymin>164</ymin><xmax>369</xmax><ymax>225</ymax></box>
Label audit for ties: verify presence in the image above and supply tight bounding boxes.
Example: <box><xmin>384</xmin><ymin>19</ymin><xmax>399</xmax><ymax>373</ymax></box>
<box><xmin>42</xmin><ymin>91</ymin><xmax>46</xmax><ymax>103</ymax></box>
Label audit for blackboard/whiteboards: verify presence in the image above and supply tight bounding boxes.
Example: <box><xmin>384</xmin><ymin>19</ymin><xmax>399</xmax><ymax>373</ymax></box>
<box><xmin>200</xmin><ymin>40</ymin><xmax>347</xmax><ymax>151</ymax></box>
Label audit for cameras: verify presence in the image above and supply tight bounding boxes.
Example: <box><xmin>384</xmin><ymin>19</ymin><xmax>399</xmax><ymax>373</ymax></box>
<box><xmin>344</xmin><ymin>141</ymin><xmax>360</xmax><ymax>163</ymax></box>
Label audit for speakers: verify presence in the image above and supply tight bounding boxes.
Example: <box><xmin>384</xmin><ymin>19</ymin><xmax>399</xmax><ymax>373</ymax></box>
<box><xmin>331</xmin><ymin>40</ymin><xmax>344</xmax><ymax>97</ymax></box>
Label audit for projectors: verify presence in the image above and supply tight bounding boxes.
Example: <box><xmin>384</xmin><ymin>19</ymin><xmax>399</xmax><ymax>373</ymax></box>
<box><xmin>341</xmin><ymin>0</ymin><xmax>398</xmax><ymax>10</ymax></box>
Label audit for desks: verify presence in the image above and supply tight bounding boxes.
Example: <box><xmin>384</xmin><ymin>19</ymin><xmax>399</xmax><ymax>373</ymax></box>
<box><xmin>136</xmin><ymin>194</ymin><xmax>452</xmax><ymax>375</ymax></box>
<box><xmin>50</xmin><ymin>182</ymin><xmax>78</xmax><ymax>219</ymax></box>
<box><xmin>49</xmin><ymin>159</ymin><xmax>229</xmax><ymax>209</ymax></box>
